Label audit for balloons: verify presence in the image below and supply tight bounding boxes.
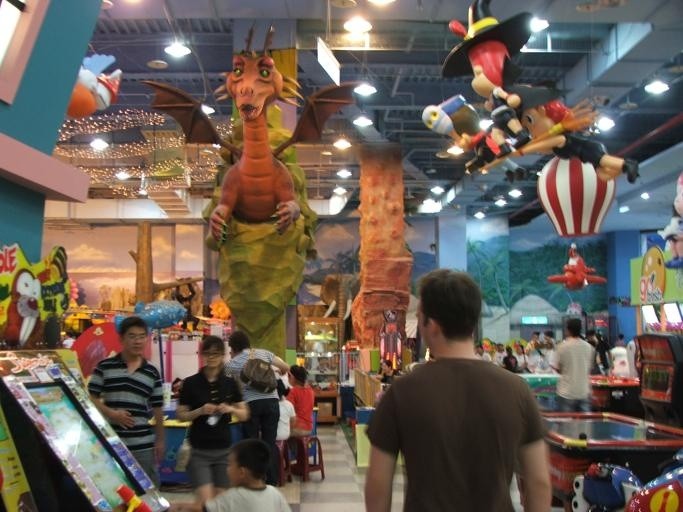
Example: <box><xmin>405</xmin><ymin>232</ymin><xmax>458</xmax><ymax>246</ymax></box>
<box><xmin>572</xmin><ymin>449</ymin><xmax>682</xmax><ymax>512</ymax></box>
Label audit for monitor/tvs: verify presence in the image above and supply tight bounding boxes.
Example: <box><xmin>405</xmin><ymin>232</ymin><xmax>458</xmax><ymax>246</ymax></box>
<box><xmin>22</xmin><ymin>377</ymin><xmax>147</xmax><ymax>510</ymax></box>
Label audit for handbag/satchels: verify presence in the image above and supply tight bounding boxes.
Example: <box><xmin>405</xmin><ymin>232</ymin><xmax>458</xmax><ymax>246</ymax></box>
<box><xmin>240</xmin><ymin>358</ymin><xmax>279</xmax><ymax>393</ymax></box>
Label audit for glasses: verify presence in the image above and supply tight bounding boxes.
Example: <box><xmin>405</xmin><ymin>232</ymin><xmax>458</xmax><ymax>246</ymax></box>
<box><xmin>203</xmin><ymin>352</ymin><xmax>220</xmax><ymax>357</ymax></box>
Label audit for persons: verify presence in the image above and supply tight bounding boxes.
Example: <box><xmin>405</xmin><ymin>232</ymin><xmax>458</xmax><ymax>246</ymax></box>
<box><xmin>379</xmin><ymin>360</ymin><xmax>400</xmax><ymax>382</ymax></box>
<box><xmin>366</xmin><ymin>268</ymin><xmax>551</xmax><ymax>511</ymax></box>
<box><xmin>286</xmin><ymin>364</ymin><xmax>314</xmax><ymax>439</ymax></box>
<box><xmin>167</xmin><ymin>439</ymin><xmax>292</xmax><ymax>512</ymax></box>
<box><xmin>474</xmin><ymin>321</ymin><xmax>629</xmax><ymax>376</ymax></box>
<box><xmin>275</xmin><ymin>380</ymin><xmax>296</xmax><ymax>450</ymax></box>
<box><xmin>84</xmin><ymin>316</ymin><xmax>164</xmax><ymax>493</ymax></box>
<box><xmin>174</xmin><ymin>335</ymin><xmax>250</xmax><ymax>502</ymax></box>
<box><xmin>223</xmin><ymin>332</ymin><xmax>289</xmax><ymax>444</ymax></box>
<box><xmin>551</xmin><ymin>319</ymin><xmax>596</xmax><ymax>401</ymax></box>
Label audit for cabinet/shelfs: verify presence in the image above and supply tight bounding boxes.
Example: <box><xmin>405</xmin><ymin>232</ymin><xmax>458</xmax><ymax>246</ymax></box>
<box><xmin>304</xmin><ymin>317</ymin><xmax>344</xmax><ymax>425</ymax></box>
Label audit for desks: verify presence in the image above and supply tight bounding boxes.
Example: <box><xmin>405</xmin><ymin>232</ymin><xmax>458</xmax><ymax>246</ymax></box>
<box><xmin>541</xmin><ymin>412</ymin><xmax>683</xmax><ymax>512</ymax></box>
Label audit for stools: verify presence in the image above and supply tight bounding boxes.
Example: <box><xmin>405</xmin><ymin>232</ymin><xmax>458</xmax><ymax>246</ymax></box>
<box><xmin>275</xmin><ymin>436</ymin><xmax>325</xmax><ymax>486</ymax></box>
